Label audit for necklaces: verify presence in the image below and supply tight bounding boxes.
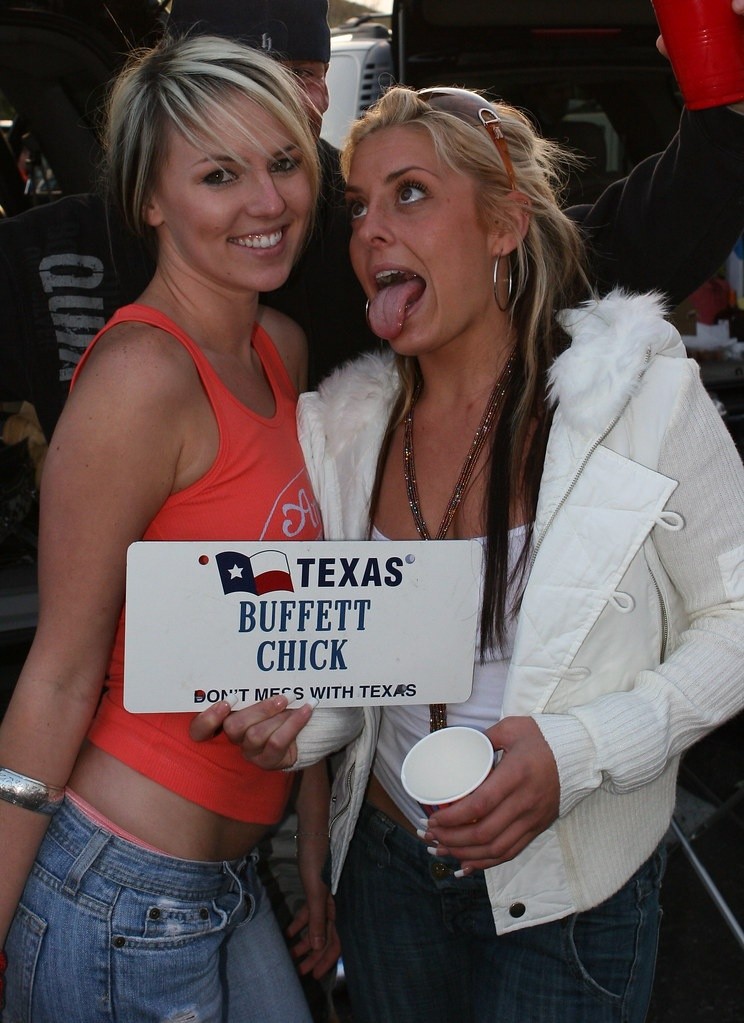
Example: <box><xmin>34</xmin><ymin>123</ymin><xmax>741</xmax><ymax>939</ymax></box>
<box><xmin>402</xmin><ymin>332</ymin><xmax>520</xmax><ymax>732</ymax></box>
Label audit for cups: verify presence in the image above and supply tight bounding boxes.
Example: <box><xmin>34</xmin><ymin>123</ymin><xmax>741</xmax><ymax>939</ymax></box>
<box><xmin>401</xmin><ymin>726</ymin><xmax>496</xmax><ymax>820</ymax></box>
<box><xmin>652</xmin><ymin>0</ymin><xmax>744</xmax><ymax>110</ymax></box>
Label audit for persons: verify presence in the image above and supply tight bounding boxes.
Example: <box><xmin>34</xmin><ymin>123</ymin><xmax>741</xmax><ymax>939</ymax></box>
<box><xmin>0</xmin><ymin>29</ymin><xmax>342</xmax><ymax>1023</ymax></box>
<box><xmin>0</xmin><ymin>0</ymin><xmax>744</xmax><ymax>456</ymax></box>
<box><xmin>191</xmin><ymin>80</ymin><xmax>744</xmax><ymax>1023</ymax></box>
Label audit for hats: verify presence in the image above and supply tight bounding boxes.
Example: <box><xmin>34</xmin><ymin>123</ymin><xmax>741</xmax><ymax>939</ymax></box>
<box><xmin>167</xmin><ymin>0</ymin><xmax>331</xmax><ymax>63</ymax></box>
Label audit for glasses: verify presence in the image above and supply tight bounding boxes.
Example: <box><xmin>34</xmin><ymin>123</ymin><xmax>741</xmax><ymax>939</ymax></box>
<box><xmin>414</xmin><ymin>86</ymin><xmax>515</xmax><ymax>189</ymax></box>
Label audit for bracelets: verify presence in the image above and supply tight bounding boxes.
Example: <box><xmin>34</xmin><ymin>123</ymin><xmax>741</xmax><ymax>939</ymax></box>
<box><xmin>287</xmin><ymin>833</ymin><xmax>331</xmax><ymax>858</ymax></box>
<box><xmin>0</xmin><ymin>767</ymin><xmax>64</xmax><ymax>816</ymax></box>
